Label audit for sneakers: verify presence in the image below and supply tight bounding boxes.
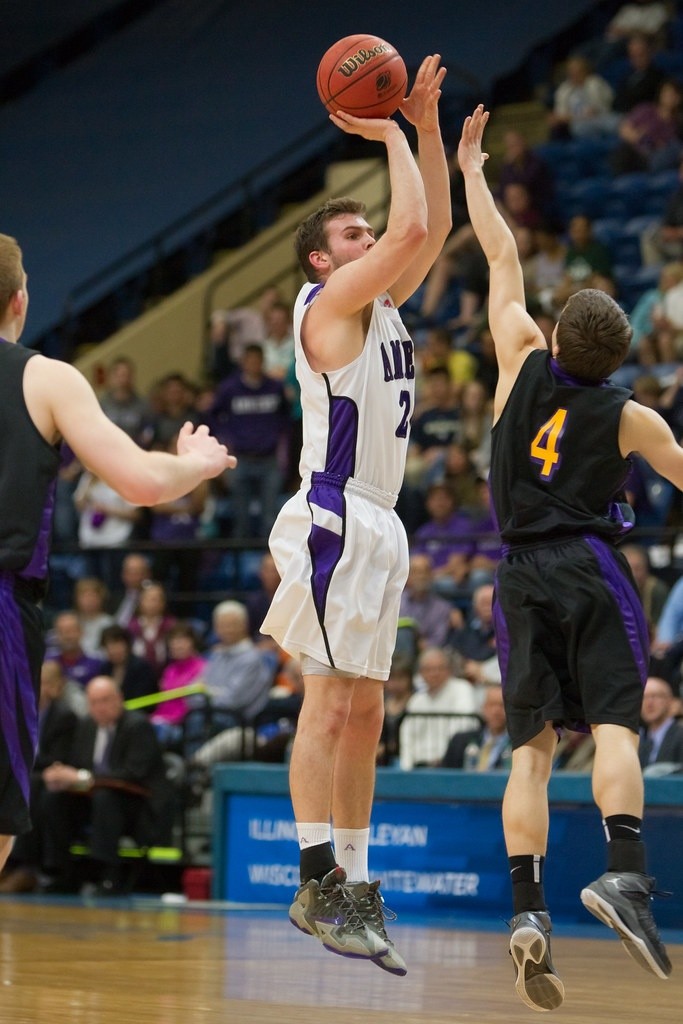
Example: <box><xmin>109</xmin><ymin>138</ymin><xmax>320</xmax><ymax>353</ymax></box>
<box><xmin>344</xmin><ymin>880</ymin><xmax>407</xmax><ymax>977</ymax></box>
<box><xmin>289</xmin><ymin>866</ymin><xmax>388</xmax><ymax>959</ymax></box>
<box><xmin>509</xmin><ymin>910</ymin><xmax>564</xmax><ymax>1011</ymax></box>
<box><xmin>580</xmin><ymin>871</ymin><xmax>673</xmax><ymax>979</ymax></box>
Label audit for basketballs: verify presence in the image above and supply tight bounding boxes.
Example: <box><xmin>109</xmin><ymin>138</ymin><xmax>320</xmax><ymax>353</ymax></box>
<box><xmin>316</xmin><ymin>34</ymin><xmax>408</xmax><ymax>122</ymax></box>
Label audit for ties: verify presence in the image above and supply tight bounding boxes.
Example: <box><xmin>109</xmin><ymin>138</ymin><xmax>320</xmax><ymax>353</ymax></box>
<box><xmin>99</xmin><ymin>728</ymin><xmax>113</xmax><ymax>774</ymax></box>
<box><xmin>639</xmin><ymin>736</ymin><xmax>652</xmax><ymax>769</ymax></box>
<box><xmin>478</xmin><ymin>738</ymin><xmax>493</xmax><ymax>770</ymax></box>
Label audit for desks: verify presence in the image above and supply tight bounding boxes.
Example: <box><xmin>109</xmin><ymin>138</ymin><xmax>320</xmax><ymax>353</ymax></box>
<box><xmin>211</xmin><ymin>762</ymin><xmax>683</xmax><ymax>945</ymax></box>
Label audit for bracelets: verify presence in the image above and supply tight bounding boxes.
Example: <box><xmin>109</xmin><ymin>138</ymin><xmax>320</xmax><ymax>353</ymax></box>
<box><xmin>77</xmin><ymin>768</ymin><xmax>94</xmax><ymax>785</ymax></box>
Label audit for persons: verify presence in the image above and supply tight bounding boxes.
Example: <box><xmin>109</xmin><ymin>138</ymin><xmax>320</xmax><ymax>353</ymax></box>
<box><xmin>0</xmin><ymin>231</ymin><xmax>237</xmax><ymax>869</ymax></box>
<box><xmin>260</xmin><ymin>54</ymin><xmax>453</xmax><ymax>975</ymax></box>
<box><xmin>0</xmin><ymin>1</ymin><xmax>683</xmax><ymax>902</ymax></box>
<box><xmin>38</xmin><ymin>35</ymin><xmax>683</xmax><ymax>902</ymax></box>
<box><xmin>456</xmin><ymin>103</ymin><xmax>683</xmax><ymax>1014</ymax></box>
